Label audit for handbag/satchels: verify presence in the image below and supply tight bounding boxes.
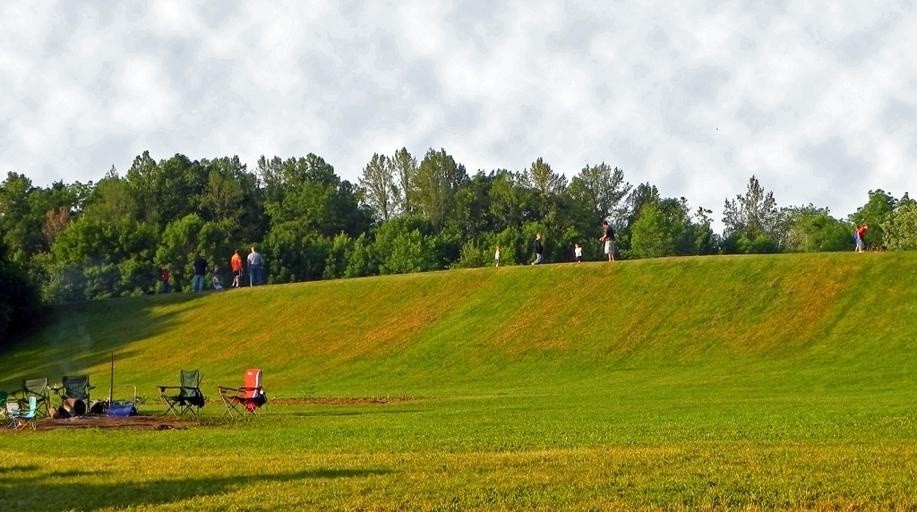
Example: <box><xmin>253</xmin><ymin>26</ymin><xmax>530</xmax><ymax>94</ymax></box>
<box><xmin>106</xmin><ymin>405</ymin><xmax>138</xmax><ymax>416</ymax></box>
<box><xmin>253</xmin><ymin>389</ymin><xmax>268</xmax><ymax>408</ymax></box>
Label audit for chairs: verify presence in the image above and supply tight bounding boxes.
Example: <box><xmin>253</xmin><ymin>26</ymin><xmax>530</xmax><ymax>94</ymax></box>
<box><xmin>157</xmin><ymin>370</ymin><xmax>204</xmax><ymax>418</ymax></box>
<box><xmin>0</xmin><ymin>375</ymin><xmax>146</xmax><ymax>431</ymax></box>
<box><xmin>218</xmin><ymin>369</ymin><xmax>264</xmax><ymax>420</ymax></box>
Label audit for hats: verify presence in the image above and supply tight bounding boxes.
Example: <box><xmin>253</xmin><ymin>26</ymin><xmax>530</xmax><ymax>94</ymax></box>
<box><xmin>601</xmin><ymin>221</ymin><xmax>607</xmax><ymax>225</ymax></box>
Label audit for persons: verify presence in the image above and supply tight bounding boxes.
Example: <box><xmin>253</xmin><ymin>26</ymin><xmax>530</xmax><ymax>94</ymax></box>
<box><xmin>574</xmin><ymin>243</ymin><xmax>583</xmax><ymax>263</ymax></box>
<box><xmin>211</xmin><ymin>264</ymin><xmax>224</xmax><ymax>291</ymax></box>
<box><xmin>598</xmin><ymin>220</ymin><xmax>615</xmax><ymax>263</ymax></box>
<box><xmin>531</xmin><ymin>232</ymin><xmax>544</xmax><ymax>265</ymax></box>
<box><xmin>855</xmin><ymin>223</ymin><xmax>869</xmax><ymax>252</ymax></box>
<box><xmin>494</xmin><ymin>245</ymin><xmax>501</xmax><ymax>268</ymax></box>
<box><xmin>229</xmin><ymin>248</ymin><xmax>243</xmax><ymax>289</ymax></box>
<box><xmin>245</xmin><ymin>246</ymin><xmax>266</xmax><ymax>289</ymax></box>
<box><xmin>160</xmin><ymin>266</ymin><xmax>168</xmax><ymax>294</ymax></box>
<box><xmin>853</xmin><ymin>225</ymin><xmax>864</xmax><ymax>252</ymax></box>
<box><xmin>191</xmin><ymin>254</ymin><xmax>207</xmax><ymax>292</ymax></box>
